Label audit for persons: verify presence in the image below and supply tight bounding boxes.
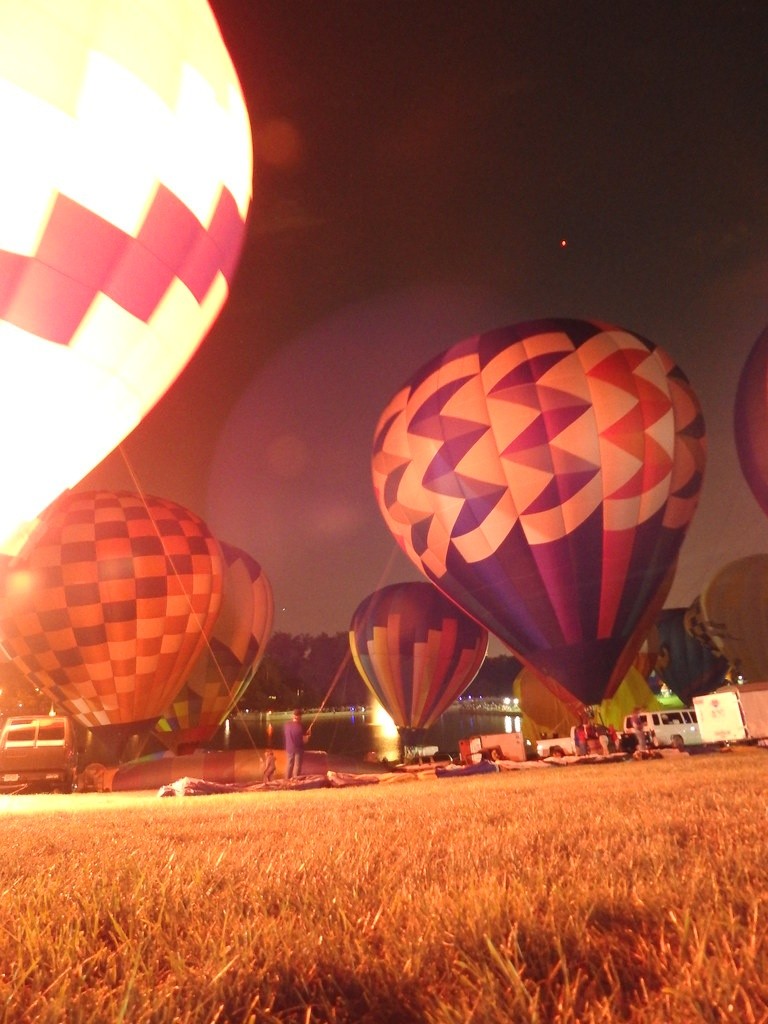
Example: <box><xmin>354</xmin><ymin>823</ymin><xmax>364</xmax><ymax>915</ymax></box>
<box><xmin>283</xmin><ymin>709</ymin><xmax>311</xmax><ymax>780</ymax></box>
<box><xmin>263</xmin><ymin>750</ymin><xmax>276</xmax><ymax>787</ymax></box>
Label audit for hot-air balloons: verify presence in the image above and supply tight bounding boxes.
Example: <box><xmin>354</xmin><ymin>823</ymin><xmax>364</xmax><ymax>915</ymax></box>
<box><xmin>0</xmin><ymin>0</ymin><xmax>258</xmax><ymax>561</ymax></box>
<box><xmin>148</xmin><ymin>541</ymin><xmax>278</xmax><ymax>757</ymax></box>
<box><xmin>367</xmin><ymin>318</ymin><xmax>705</xmax><ymax>755</ymax></box>
<box><xmin>697</xmin><ymin>553</ymin><xmax>767</xmax><ymax>750</ymax></box>
<box><xmin>2</xmin><ymin>491</ymin><xmax>224</xmax><ymax>767</ymax></box>
<box><xmin>348</xmin><ymin>582</ymin><xmax>492</xmax><ymax>766</ymax></box>
<box><xmin>734</xmin><ymin>322</ymin><xmax>768</xmax><ymax>522</ymax></box>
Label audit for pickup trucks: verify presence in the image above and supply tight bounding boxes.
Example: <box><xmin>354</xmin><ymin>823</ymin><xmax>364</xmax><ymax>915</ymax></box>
<box><xmin>534</xmin><ymin>724</ymin><xmax>623</xmax><ymax>760</ymax></box>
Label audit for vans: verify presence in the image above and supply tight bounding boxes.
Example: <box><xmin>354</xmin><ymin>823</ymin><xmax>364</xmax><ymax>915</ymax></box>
<box><xmin>622</xmin><ymin>710</ymin><xmax>702</xmax><ymax>748</ymax></box>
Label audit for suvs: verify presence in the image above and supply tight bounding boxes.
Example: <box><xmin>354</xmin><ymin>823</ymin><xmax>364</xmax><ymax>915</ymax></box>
<box><xmin>0</xmin><ymin>713</ymin><xmax>87</xmax><ymax>794</ymax></box>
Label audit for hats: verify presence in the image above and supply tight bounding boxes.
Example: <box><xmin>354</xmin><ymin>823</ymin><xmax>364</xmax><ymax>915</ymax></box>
<box><xmin>293</xmin><ymin>709</ymin><xmax>304</xmax><ymax>715</ymax></box>
